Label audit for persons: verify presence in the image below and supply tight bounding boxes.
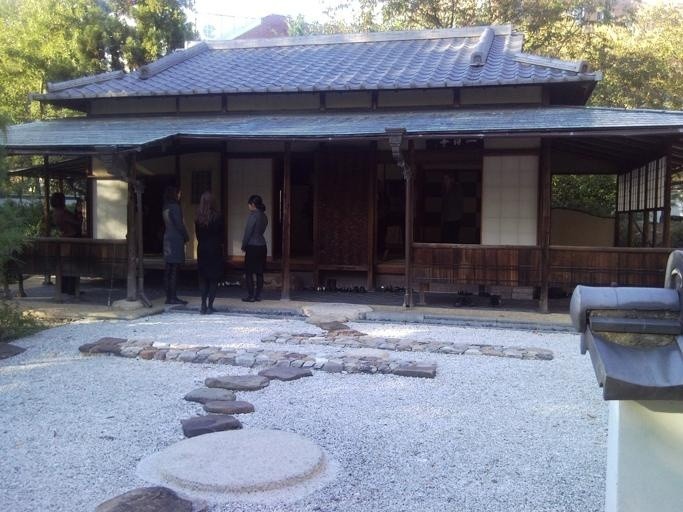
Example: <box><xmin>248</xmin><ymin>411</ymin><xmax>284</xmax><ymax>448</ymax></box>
<box><xmin>239</xmin><ymin>195</ymin><xmax>270</xmax><ymax>302</ymax></box>
<box><xmin>48</xmin><ymin>192</ymin><xmax>83</xmax><ymax>296</ymax></box>
<box><xmin>194</xmin><ymin>191</ymin><xmax>226</xmax><ymax>313</ymax></box>
<box><xmin>73</xmin><ymin>199</ymin><xmax>87</xmax><ymax>223</ymax></box>
<box><xmin>159</xmin><ymin>184</ymin><xmax>189</xmax><ymax>304</ymax></box>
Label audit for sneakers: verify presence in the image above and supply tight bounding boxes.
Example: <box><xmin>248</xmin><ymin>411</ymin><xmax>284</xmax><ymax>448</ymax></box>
<box><xmin>253</xmin><ymin>296</ymin><xmax>261</xmax><ymax>301</ymax></box>
<box><xmin>241</xmin><ymin>296</ymin><xmax>254</xmax><ymax>302</ymax></box>
<box><xmin>199</xmin><ymin>307</ymin><xmax>219</xmax><ymax>315</ymax></box>
<box><xmin>164</xmin><ymin>298</ymin><xmax>188</xmax><ymax>305</ymax></box>
